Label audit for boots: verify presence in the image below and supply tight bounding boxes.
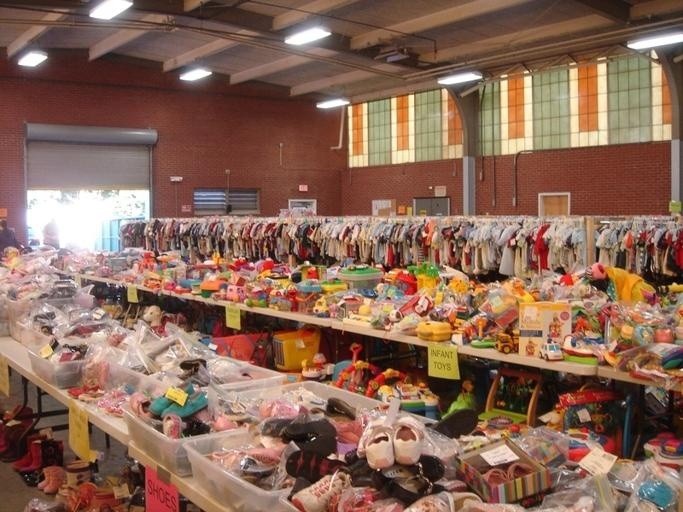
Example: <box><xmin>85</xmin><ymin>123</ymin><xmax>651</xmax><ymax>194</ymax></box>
<box><xmin>1</xmin><ymin>404</ymin><xmax>150</xmax><ymax>512</ymax></box>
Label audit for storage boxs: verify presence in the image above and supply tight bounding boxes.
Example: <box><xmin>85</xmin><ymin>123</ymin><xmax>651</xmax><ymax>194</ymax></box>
<box><xmin>0</xmin><ymin>268</ymin><xmax>683</xmax><ymax>512</ymax></box>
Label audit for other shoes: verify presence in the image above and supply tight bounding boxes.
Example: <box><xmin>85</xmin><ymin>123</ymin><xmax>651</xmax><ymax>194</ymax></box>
<box><xmin>66</xmin><ymin>376</ymin><xmax>238</xmax><ymax>441</ymax></box>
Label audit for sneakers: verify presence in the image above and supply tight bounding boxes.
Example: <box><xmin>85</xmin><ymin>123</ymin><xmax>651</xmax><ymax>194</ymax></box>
<box><xmin>234</xmin><ymin>388</ymin><xmax>541</xmax><ymax>512</ymax></box>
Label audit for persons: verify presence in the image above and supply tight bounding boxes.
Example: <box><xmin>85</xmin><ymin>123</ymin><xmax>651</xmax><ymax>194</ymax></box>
<box><xmin>0</xmin><ymin>217</ymin><xmax>60</xmax><ymax>257</ymax></box>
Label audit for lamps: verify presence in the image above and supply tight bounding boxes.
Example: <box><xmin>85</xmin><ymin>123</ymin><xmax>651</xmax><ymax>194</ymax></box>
<box><xmin>179</xmin><ymin>59</ymin><xmax>213</xmax><ymax>84</ymax></box>
<box><xmin>282</xmin><ymin>14</ymin><xmax>332</xmax><ymax>47</ymax></box>
<box><xmin>17</xmin><ymin>39</ymin><xmax>48</xmax><ymax>68</ymax></box>
<box><xmin>313</xmin><ymin>97</ymin><xmax>351</xmax><ymax>110</ymax></box>
<box><xmin>438</xmin><ymin>69</ymin><xmax>484</xmax><ymax>85</ymax></box>
<box><xmin>89</xmin><ymin>0</ymin><xmax>134</xmax><ymax>22</ymax></box>
<box><xmin>627</xmin><ymin>31</ymin><xmax>683</xmax><ymax>52</ymax></box>
